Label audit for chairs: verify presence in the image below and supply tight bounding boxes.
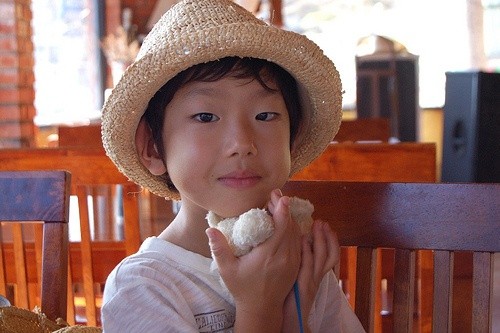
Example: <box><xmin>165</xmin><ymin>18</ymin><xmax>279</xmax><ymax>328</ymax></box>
<box><xmin>330</xmin><ymin>119</ymin><xmax>391</xmax><ymax>143</ymax></box>
<box><xmin>264</xmin><ymin>180</ymin><xmax>499</xmax><ymax>333</ymax></box>
<box><xmin>57</xmin><ymin>125</ymin><xmax>155</xmax><ymax>241</ymax></box>
<box><xmin>0</xmin><ymin>170</ymin><xmax>71</xmax><ymax>326</ymax></box>
<box><xmin>288</xmin><ymin>141</ymin><xmax>437</xmax><ymax>333</ymax></box>
<box><xmin>0</xmin><ymin>146</ymin><xmax>140</xmax><ymax>328</ymax></box>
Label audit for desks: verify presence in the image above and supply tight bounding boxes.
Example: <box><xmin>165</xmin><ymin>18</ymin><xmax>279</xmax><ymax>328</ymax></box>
<box><xmin>2</xmin><ymin>192</ymin><xmax>175</xmax><ymax>281</ymax></box>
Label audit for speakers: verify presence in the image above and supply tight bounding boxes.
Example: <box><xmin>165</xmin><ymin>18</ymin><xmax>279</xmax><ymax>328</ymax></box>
<box><xmin>441</xmin><ymin>70</ymin><xmax>500</xmax><ymax>184</ymax></box>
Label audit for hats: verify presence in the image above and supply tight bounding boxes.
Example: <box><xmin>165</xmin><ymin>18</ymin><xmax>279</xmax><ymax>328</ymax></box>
<box><xmin>101</xmin><ymin>0</ymin><xmax>343</xmax><ymax>201</ymax></box>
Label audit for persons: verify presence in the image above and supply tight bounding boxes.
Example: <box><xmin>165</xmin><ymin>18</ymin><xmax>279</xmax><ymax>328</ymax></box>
<box><xmin>101</xmin><ymin>1</ymin><xmax>367</xmax><ymax>333</ymax></box>
